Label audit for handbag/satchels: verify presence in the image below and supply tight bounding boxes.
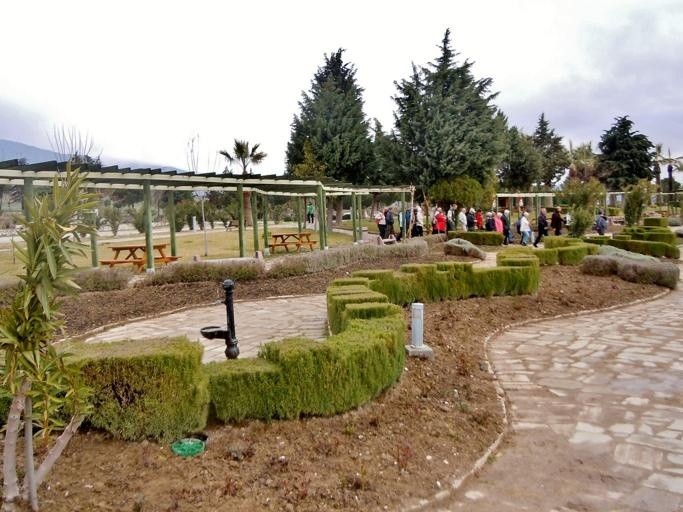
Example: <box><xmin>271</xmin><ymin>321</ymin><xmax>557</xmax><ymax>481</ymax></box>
<box><xmin>374</xmin><ymin>219</ymin><xmax>380</xmax><ymax>224</ymax></box>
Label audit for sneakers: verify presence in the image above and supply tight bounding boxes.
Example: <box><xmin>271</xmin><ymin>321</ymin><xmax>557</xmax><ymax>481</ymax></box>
<box><xmin>503</xmin><ymin>242</ymin><xmax>538</xmax><ymax>247</ymax></box>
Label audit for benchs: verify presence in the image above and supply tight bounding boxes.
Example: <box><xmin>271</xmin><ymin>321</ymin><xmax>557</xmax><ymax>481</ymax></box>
<box><xmin>377</xmin><ymin>234</ymin><xmax>397</xmax><ymax>246</ymax></box>
<box><xmin>269</xmin><ymin>242</ymin><xmax>303</xmax><ymax>253</ymax></box>
<box><xmin>286</xmin><ymin>240</ymin><xmax>317</xmax><ymax>251</ymax></box>
<box><xmin>99</xmin><ymin>258</ymin><xmax>147</xmax><ymax>275</ymax></box>
<box><xmin>152</xmin><ymin>255</ymin><xmax>182</xmax><ymax>262</ymax></box>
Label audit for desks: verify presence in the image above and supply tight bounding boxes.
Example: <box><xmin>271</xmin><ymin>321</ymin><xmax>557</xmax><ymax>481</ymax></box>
<box><xmin>107</xmin><ymin>242</ymin><xmax>169</xmax><ymax>266</ymax></box>
<box><xmin>271</xmin><ymin>232</ymin><xmax>312</xmax><ymax>251</ymax></box>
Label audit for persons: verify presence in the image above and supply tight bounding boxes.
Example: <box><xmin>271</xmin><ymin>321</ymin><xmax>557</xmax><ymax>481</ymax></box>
<box><xmin>592</xmin><ymin>208</ymin><xmax>608</xmax><ymax>235</ymax></box>
<box><xmin>519</xmin><ymin>197</ymin><xmax>524</xmax><ymax>211</ymax></box>
<box><xmin>375</xmin><ymin>204</ymin><xmax>571</xmax><ymax>247</ymax></box>
<box><xmin>307</xmin><ymin>202</ymin><xmax>314</xmax><ymax>223</ymax></box>
